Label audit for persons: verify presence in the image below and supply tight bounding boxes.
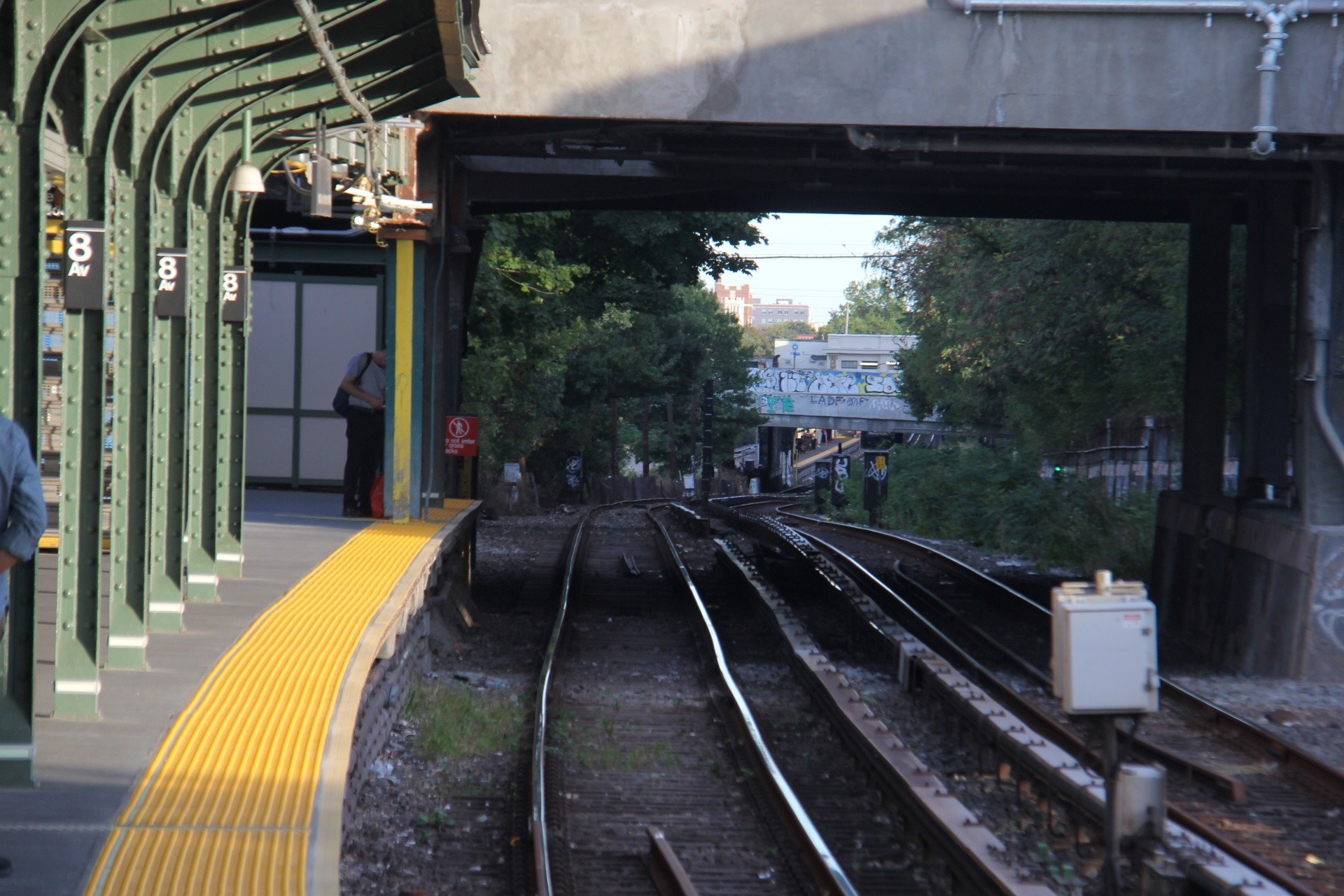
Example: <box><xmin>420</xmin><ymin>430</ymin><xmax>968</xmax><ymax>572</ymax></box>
<box><xmin>811</xmin><ymin>430</ymin><xmax>821</xmax><ymax>449</ymax></box>
<box><xmin>822</xmin><ymin>429</ymin><xmax>829</xmax><ymax>442</ymax></box>
<box><xmin>339</xmin><ymin>342</ymin><xmax>388</xmax><ymax>518</ymax></box>
<box><xmin>0</xmin><ymin>411</ymin><xmax>48</xmax><ymax>644</ymax></box>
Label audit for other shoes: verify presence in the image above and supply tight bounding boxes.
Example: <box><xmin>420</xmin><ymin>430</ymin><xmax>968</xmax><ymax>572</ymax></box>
<box><xmin>342</xmin><ymin>504</ymin><xmax>364</xmax><ymax>517</ymax></box>
<box><xmin>360</xmin><ymin>501</ymin><xmax>372</xmax><ymax>516</ymax></box>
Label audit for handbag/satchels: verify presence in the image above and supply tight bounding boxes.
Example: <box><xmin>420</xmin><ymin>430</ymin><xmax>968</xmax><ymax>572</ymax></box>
<box><xmin>332</xmin><ymin>352</ymin><xmax>372</xmax><ymax>418</ymax></box>
<box><xmin>369</xmin><ymin>474</ymin><xmax>385</xmax><ymax>519</ymax></box>
